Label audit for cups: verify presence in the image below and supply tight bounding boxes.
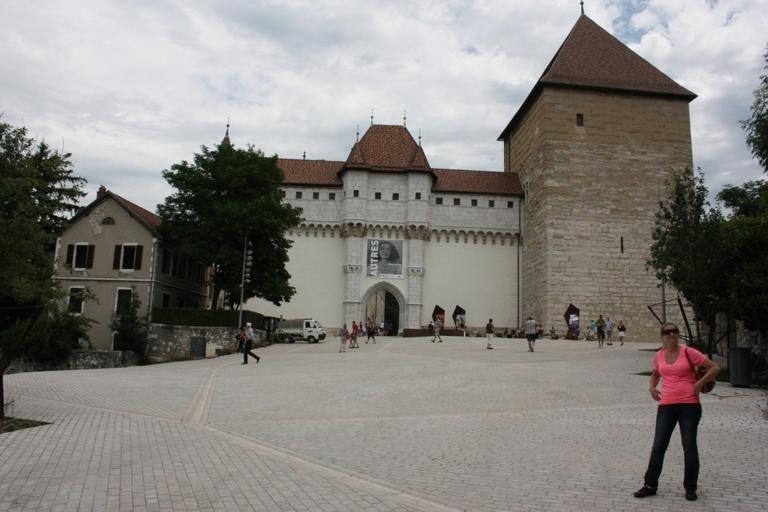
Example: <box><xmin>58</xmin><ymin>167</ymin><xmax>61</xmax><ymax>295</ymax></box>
<box><xmin>663</xmin><ymin>329</ymin><xmax>679</xmax><ymax>334</ymax></box>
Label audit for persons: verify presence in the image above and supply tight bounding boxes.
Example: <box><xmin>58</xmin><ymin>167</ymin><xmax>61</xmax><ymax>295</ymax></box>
<box><xmin>338</xmin><ymin>322</ymin><xmax>349</xmax><ymax>352</ymax></box>
<box><xmin>526</xmin><ymin>317</ymin><xmax>536</xmax><ymax>352</ymax></box>
<box><xmin>518</xmin><ymin>324</ymin><xmax>559</xmax><ymax>339</ymax></box>
<box><xmin>364</xmin><ymin>317</ymin><xmax>376</xmax><ymax>344</ymax></box>
<box><xmin>584</xmin><ymin>314</ymin><xmax>626</xmax><ymax>348</ymax></box>
<box><xmin>390</xmin><ymin>323</ymin><xmax>394</xmax><ymax>335</ymax></box>
<box><xmin>367</xmin><ymin>242</ymin><xmax>400</xmax><ymax>274</ymax></box>
<box><xmin>237</xmin><ymin>326</ymin><xmax>245</xmax><ymax>352</ymax></box>
<box><xmin>431</xmin><ymin>317</ymin><xmax>442</xmax><ymax>342</ymax></box>
<box><xmin>348</xmin><ymin>333</ymin><xmax>354</xmax><ymax>348</ymax></box>
<box><xmin>632</xmin><ymin>322</ymin><xmax>720</xmax><ymax>500</ymax></box>
<box><xmin>379</xmin><ymin>320</ymin><xmax>385</xmax><ymax>334</ymax></box>
<box><xmin>359</xmin><ymin>321</ymin><xmax>362</xmax><ymax>332</ymax></box>
<box><xmin>242</xmin><ymin>321</ymin><xmax>261</xmax><ymax>365</ymax></box>
<box><xmin>385</xmin><ymin>319</ymin><xmax>389</xmax><ymax>336</ymax></box>
<box><xmin>352</xmin><ymin>320</ymin><xmax>359</xmax><ymax>348</ymax></box>
<box><xmin>429</xmin><ymin>321</ymin><xmax>434</xmax><ymax>333</ymax></box>
<box><xmin>485</xmin><ymin>318</ymin><xmax>495</xmax><ymax>350</ymax></box>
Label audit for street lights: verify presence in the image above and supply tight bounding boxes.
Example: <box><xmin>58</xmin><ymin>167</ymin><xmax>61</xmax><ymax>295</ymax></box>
<box><xmin>695</xmin><ymin>366</ymin><xmax>713</xmax><ymax>393</ymax></box>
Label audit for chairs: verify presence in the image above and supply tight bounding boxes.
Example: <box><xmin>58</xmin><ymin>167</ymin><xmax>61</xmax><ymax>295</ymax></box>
<box><xmin>685</xmin><ymin>490</ymin><xmax>696</xmax><ymax>501</ymax></box>
<box><xmin>634</xmin><ymin>487</ymin><xmax>655</xmax><ymax>498</ymax></box>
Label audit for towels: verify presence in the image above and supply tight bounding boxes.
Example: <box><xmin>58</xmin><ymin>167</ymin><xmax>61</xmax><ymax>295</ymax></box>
<box><xmin>189</xmin><ymin>336</ymin><xmax>205</xmax><ymax>360</ymax></box>
<box><xmin>727</xmin><ymin>348</ymin><xmax>752</xmax><ymax>386</ymax></box>
